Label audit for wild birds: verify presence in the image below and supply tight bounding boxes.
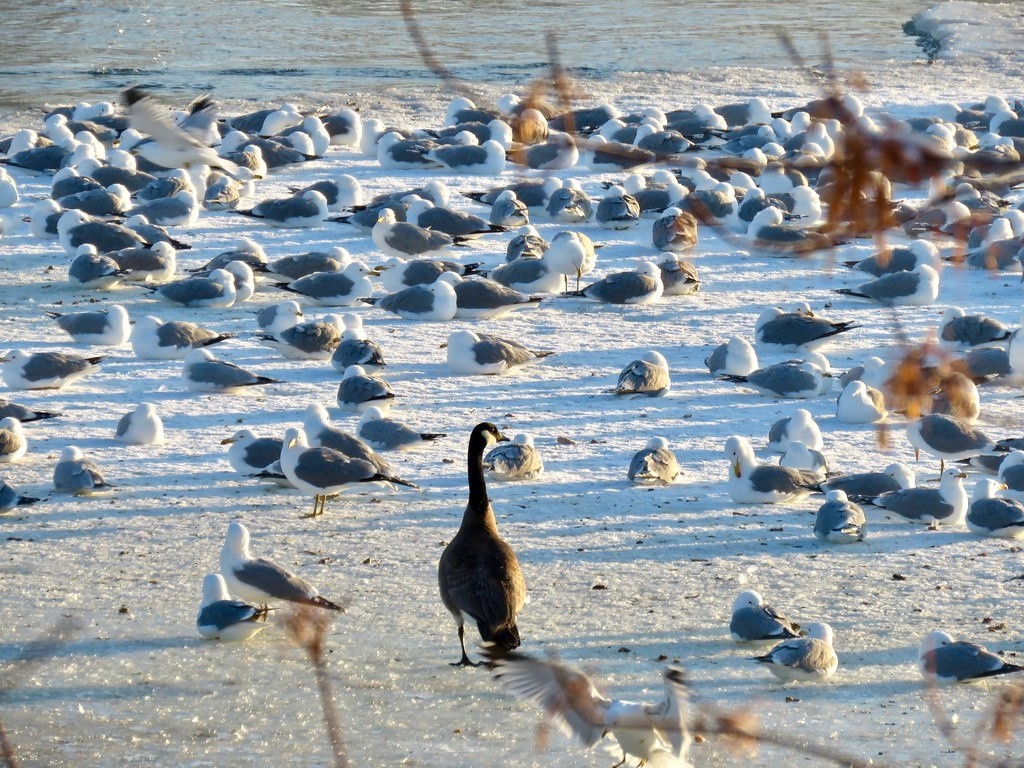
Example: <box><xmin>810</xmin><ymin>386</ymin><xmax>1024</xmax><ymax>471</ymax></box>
<box><xmin>0</xmin><ymin>82</ymin><xmax>1024</xmax><ymax>768</ymax></box>
